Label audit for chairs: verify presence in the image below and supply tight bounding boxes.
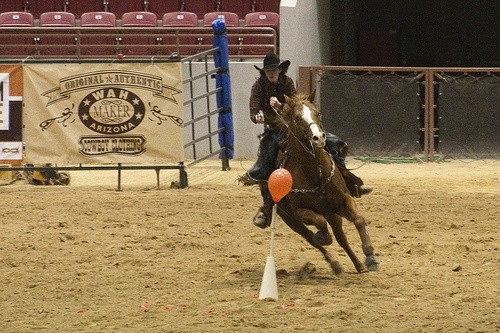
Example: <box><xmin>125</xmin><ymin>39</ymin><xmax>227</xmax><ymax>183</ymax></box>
<box><xmin>0</xmin><ymin>0</ymin><xmax>280</xmax><ymax>55</ymax></box>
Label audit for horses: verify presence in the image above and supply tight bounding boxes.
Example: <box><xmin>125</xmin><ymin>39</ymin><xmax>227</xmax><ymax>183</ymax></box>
<box><xmin>267</xmin><ymin>87</ymin><xmax>379</xmax><ymax>277</ymax></box>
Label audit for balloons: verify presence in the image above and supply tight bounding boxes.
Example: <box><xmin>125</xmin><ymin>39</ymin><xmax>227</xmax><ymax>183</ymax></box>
<box><xmin>267</xmin><ymin>168</ymin><xmax>293</xmax><ymax>204</ymax></box>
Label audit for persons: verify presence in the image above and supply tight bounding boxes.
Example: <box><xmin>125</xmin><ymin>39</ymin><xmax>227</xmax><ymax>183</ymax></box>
<box><xmin>249</xmin><ymin>51</ymin><xmax>373</xmax><ymax>229</ymax></box>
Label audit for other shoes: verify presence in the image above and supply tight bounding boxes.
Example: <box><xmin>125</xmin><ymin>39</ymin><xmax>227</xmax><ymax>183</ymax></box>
<box><xmin>360</xmin><ymin>184</ymin><xmax>374</xmax><ymax>195</ymax></box>
<box><xmin>253</xmin><ymin>209</ymin><xmax>273</xmax><ymax>229</ymax></box>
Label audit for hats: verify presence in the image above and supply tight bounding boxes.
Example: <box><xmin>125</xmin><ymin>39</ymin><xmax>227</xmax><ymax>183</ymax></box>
<box><xmin>253</xmin><ymin>53</ymin><xmax>291</xmax><ymax>76</ymax></box>
